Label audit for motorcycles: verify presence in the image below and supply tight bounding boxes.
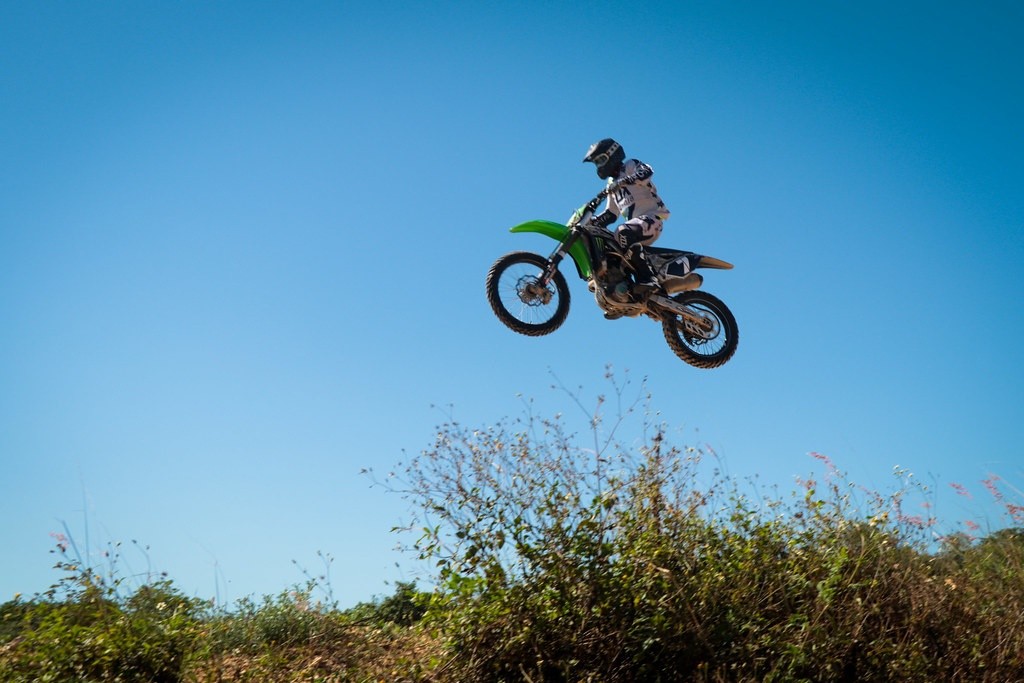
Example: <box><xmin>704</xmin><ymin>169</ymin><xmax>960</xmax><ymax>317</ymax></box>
<box><xmin>485</xmin><ymin>185</ymin><xmax>741</xmax><ymax>369</ymax></box>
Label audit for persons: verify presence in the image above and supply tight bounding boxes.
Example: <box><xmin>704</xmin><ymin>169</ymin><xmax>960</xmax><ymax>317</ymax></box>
<box><xmin>589</xmin><ymin>137</ymin><xmax>667</xmax><ymax>288</ymax></box>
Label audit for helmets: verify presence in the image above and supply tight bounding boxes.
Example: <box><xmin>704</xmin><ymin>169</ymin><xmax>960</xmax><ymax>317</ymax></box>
<box><xmin>582</xmin><ymin>138</ymin><xmax>625</xmax><ymax>179</ymax></box>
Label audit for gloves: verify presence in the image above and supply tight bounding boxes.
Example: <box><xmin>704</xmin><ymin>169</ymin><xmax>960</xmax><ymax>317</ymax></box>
<box><xmin>606</xmin><ymin>181</ymin><xmax>620</xmax><ymax>195</ymax></box>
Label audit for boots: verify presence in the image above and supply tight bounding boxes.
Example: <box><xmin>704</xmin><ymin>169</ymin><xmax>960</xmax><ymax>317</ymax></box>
<box><xmin>624</xmin><ymin>242</ymin><xmax>660</xmax><ymax>290</ymax></box>
